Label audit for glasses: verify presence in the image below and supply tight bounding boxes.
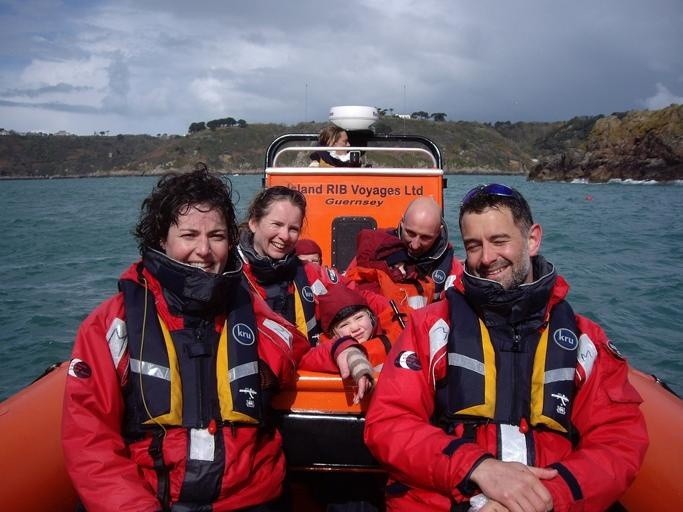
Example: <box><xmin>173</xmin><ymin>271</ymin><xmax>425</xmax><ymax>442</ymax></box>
<box><xmin>460</xmin><ymin>181</ymin><xmax>532</xmax><ymax>229</ymax></box>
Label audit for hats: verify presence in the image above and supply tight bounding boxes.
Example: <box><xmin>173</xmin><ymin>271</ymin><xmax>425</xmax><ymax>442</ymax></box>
<box><xmin>356</xmin><ymin>228</ymin><xmax>407</xmax><ymax>263</ymax></box>
<box><xmin>317</xmin><ymin>285</ymin><xmax>368</xmax><ymax>340</ymax></box>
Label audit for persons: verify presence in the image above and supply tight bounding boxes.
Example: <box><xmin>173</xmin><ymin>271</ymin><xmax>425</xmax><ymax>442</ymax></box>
<box><xmin>53</xmin><ymin>162</ymin><xmax>378</xmax><ymax>509</ymax></box>
<box><xmin>304</xmin><ymin>127</ymin><xmax>371</xmax><ymax>169</ymax></box>
<box><xmin>349</xmin><ymin>196</ymin><xmax>464</xmax><ymax>316</ymax></box>
<box><xmin>361</xmin><ymin>178</ymin><xmax>649</xmax><ymax>511</ymax></box>
<box><xmin>234</xmin><ymin>185</ymin><xmax>402</xmax><ymax>368</ymax></box>
<box><xmin>315</xmin><ymin>284</ymin><xmax>386</xmax><ymax>342</ymax></box>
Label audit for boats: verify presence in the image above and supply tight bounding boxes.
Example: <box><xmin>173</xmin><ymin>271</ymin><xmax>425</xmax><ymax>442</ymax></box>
<box><xmin>2</xmin><ymin>102</ymin><xmax>683</xmax><ymax>511</ymax></box>
